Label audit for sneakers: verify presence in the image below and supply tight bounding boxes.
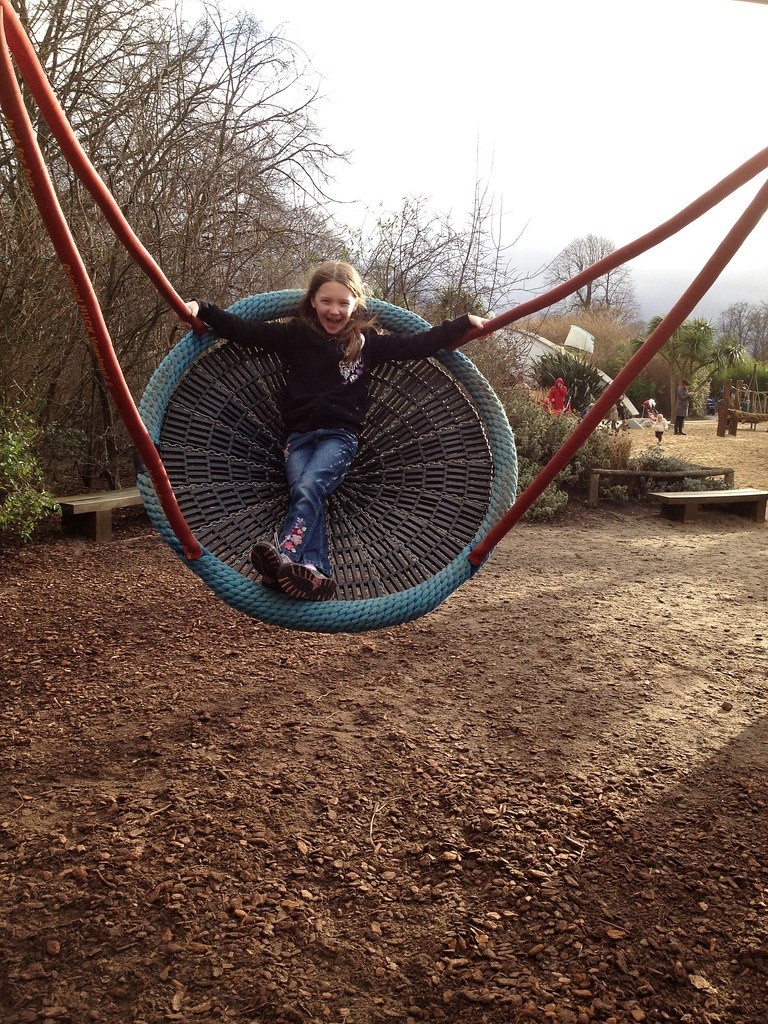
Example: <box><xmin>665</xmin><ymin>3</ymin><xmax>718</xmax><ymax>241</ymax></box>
<box><xmin>251</xmin><ymin>532</ymin><xmax>287</xmax><ymax>589</ymax></box>
<box><xmin>276</xmin><ymin>559</ymin><xmax>338</xmax><ymax>602</ymax></box>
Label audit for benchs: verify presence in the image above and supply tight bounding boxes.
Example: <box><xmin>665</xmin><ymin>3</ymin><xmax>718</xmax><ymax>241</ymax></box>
<box><xmin>648</xmin><ymin>489</ymin><xmax>768</xmax><ymax>523</ymax></box>
<box><xmin>56</xmin><ymin>486</ymin><xmax>143</xmax><ymax>542</ymax></box>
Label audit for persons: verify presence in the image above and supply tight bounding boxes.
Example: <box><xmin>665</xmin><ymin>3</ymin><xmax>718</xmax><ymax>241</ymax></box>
<box><xmin>611</xmin><ymin>395</ymin><xmax>627</xmax><ymax>430</ymax></box>
<box><xmin>674</xmin><ymin>378</ymin><xmax>694</xmax><ymax>435</ymax></box>
<box><xmin>650</xmin><ymin>414</ymin><xmax>668</xmax><ymax>446</ymax></box>
<box><xmin>549</xmin><ymin>378</ymin><xmax>571</xmax><ymax>411</ymax></box>
<box><xmin>580</xmin><ymin>403</ymin><xmax>595</xmax><ymax>418</ymax></box>
<box><xmin>174</xmin><ymin>260</ymin><xmax>492</xmax><ymax>605</ymax></box>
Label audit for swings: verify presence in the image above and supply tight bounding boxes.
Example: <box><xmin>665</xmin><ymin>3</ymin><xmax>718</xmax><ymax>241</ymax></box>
<box><xmin>0</xmin><ymin>1</ymin><xmax>768</xmax><ymax>637</ymax></box>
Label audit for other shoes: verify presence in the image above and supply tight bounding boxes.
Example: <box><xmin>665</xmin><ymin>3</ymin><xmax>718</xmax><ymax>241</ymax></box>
<box><xmin>679</xmin><ymin>431</ymin><xmax>686</xmax><ymax>435</ymax></box>
<box><xmin>674</xmin><ymin>432</ymin><xmax>681</xmax><ymax>435</ymax></box>
